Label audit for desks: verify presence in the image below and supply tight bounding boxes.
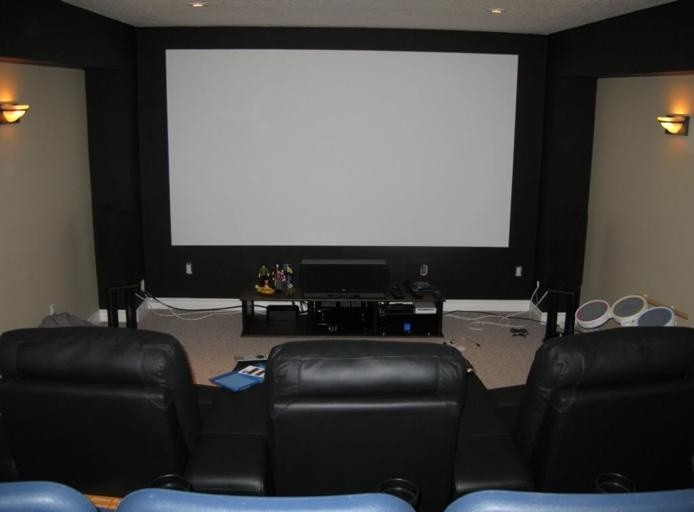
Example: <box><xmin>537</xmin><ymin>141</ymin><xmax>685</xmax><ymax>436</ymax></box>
<box><xmin>239</xmin><ymin>280</ymin><xmax>446</xmax><ymax>339</ymax></box>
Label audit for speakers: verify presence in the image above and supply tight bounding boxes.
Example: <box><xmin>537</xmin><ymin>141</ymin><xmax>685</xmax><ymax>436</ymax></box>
<box><xmin>299</xmin><ymin>259</ymin><xmax>389</xmax><ymax>299</ymax></box>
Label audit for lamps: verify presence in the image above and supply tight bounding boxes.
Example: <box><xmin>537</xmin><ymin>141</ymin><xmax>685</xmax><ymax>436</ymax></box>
<box><xmin>655</xmin><ymin>112</ymin><xmax>690</xmax><ymax>138</ymax></box>
<box><xmin>0</xmin><ymin>102</ymin><xmax>30</xmax><ymax>125</ymax></box>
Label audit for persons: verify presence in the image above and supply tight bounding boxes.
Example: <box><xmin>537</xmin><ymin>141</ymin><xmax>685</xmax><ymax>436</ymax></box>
<box><xmin>254</xmin><ymin>263</ymin><xmax>296</xmax><ymax>298</ymax></box>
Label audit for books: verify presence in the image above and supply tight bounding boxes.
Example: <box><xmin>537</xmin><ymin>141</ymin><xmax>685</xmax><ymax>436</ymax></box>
<box><xmin>208</xmin><ymin>362</ymin><xmax>266</xmax><ymax>392</ymax></box>
